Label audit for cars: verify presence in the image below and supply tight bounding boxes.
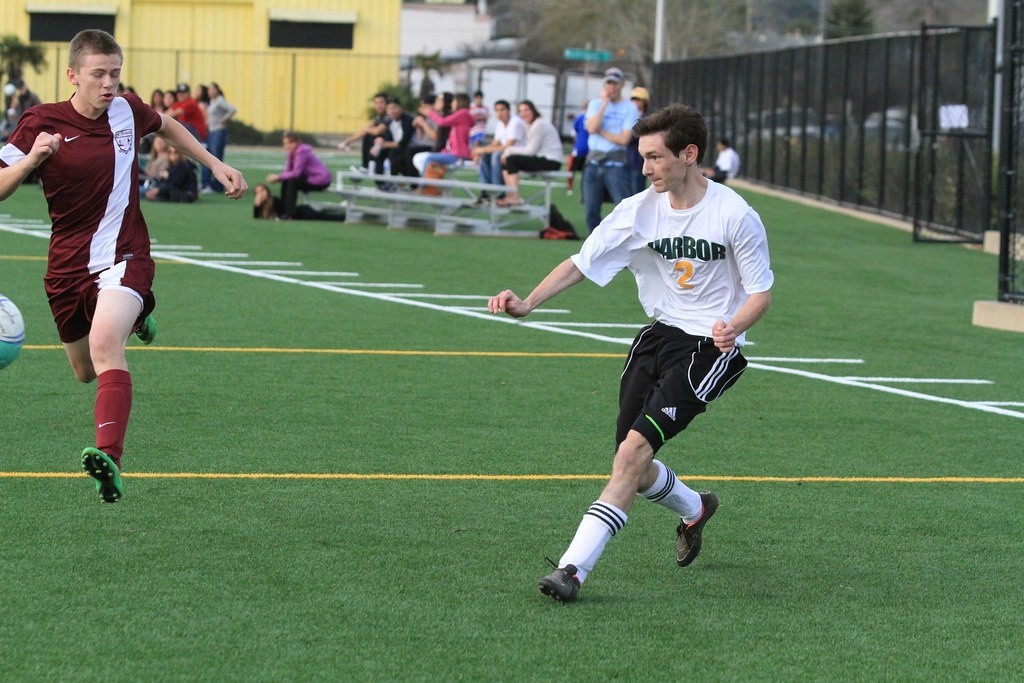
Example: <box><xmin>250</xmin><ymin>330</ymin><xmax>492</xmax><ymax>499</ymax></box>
<box><xmin>705</xmin><ymin>101</ymin><xmax>992</xmax><ymax>173</ymax></box>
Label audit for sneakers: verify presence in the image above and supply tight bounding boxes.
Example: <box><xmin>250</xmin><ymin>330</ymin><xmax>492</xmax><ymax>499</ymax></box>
<box><xmin>538</xmin><ymin>557</ymin><xmax>580</xmax><ymax>606</ymax></box>
<box><xmin>81</xmin><ymin>447</ymin><xmax>123</xmax><ymax>504</ymax></box>
<box><xmin>133</xmin><ymin>289</ymin><xmax>158</xmax><ymax>344</ymax></box>
<box><xmin>675</xmin><ymin>492</ymin><xmax>719</xmax><ymax>567</ymax></box>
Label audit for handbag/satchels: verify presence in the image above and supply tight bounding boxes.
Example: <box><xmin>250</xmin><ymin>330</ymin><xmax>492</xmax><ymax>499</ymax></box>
<box><xmin>541</xmin><ymin>205</ymin><xmax>577</xmax><ymax>239</ymax></box>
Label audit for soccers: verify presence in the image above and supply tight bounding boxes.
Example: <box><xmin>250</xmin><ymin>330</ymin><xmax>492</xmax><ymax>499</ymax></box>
<box><xmin>0</xmin><ymin>293</ymin><xmax>27</xmax><ymax>369</ymax></box>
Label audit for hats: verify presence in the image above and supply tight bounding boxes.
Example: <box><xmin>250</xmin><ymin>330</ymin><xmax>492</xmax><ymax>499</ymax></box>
<box><xmin>631</xmin><ymin>86</ymin><xmax>650</xmax><ymax>103</ymax></box>
<box><xmin>604</xmin><ymin>67</ymin><xmax>624</xmax><ymax>84</ymax></box>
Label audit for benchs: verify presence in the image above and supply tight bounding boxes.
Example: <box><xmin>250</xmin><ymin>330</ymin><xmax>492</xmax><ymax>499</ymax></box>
<box><xmin>327</xmin><ymin>158</ymin><xmax>575</xmax><ymax>239</ymax></box>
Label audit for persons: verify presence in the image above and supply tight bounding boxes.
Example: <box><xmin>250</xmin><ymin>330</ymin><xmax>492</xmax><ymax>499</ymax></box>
<box><xmin>0</xmin><ymin>81</ymin><xmax>43</xmax><ymax>183</ymax></box>
<box><xmin>567</xmin><ymin>68</ymin><xmax>639</xmax><ymax>235</ymax></box>
<box><xmin>0</xmin><ymin>30</ymin><xmax>248</xmax><ymax>503</ymax></box>
<box><xmin>702</xmin><ymin>140</ymin><xmax>740</xmax><ymax>182</ymax></box>
<box><xmin>487</xmin><ymin>104</ymin><xmax>774</xmax><ymax>605</ymax></box>
<box><xmin>471</xmin><ymin>100</ymin><xmax>564</xmax><ymax>206</ymax></box>
<box><xmin>337</xmin><ymin>93</ymin><xmax>491</xmax><ymax>198</ymax></box>
<box><xmin>627</xmin><ymin>87</ymin><xmax>652</xmax><ymax>195</ymax></box>
<box><xmin>115</xmin><ymin>83</ymin><xmax>236</xmax><ymax>203</ymax></box>
<box><xmin>254</xmin><ymin>131</ymin><xmax>345</xmax><ymax>222</ymax></box>
